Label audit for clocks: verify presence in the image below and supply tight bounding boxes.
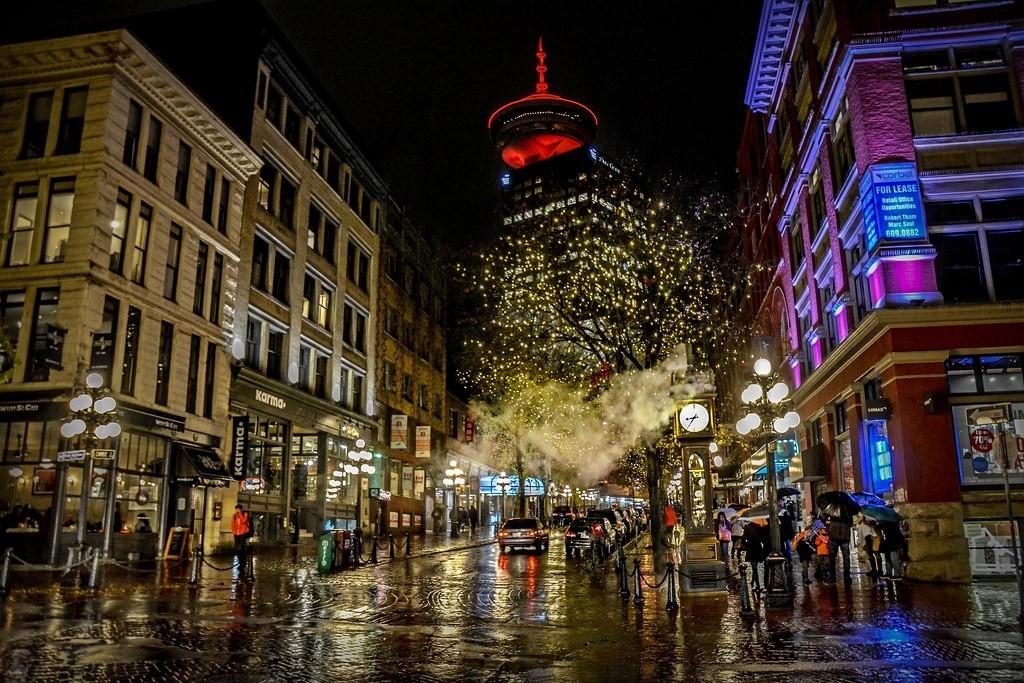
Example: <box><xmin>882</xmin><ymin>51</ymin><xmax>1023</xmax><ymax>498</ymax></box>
<box><xmin>675</xmin><ymin>398</ymin><xmax>717</xmax><ymax>437</ymax></box>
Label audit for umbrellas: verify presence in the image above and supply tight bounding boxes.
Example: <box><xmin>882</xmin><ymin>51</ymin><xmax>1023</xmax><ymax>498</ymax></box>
<box><xmin>862</xmin><ymin>504</ymin><xmax>904</xmax><ymax>523</ymax></box>
<box><xmin>816</xmin><ymin>490</ymin><xmax>861</xmax><ymax>518</ymax></box>
<box><xmin>712</xmin><ymin>499</ymin><xmax>777</xmax><ymax>526</ymax></box>
<box><xmin>777</xmin><ymin>486</ymin><xmax>800</xmax><ymax>500</ymax></box>
<box><xmin>851</xmin><ymin>491</ymin><xmax>886</xmax><ymax>507</ymax></box>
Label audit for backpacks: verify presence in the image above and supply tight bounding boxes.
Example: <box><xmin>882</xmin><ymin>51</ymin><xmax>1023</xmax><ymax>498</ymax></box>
<box><xmin>242</xmin><ymin>511</ymin><xmax>254</xmax><ymax>537</ymax></box>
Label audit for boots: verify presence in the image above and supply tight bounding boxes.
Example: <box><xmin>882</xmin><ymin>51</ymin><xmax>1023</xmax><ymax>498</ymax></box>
<box><xmin>867</xmin><ymin>558</ymin><xmax>883</xmax><ymax>574</ymax></box>
<box><xmin>880</xmin><ymin>560</ymin><xmax>893</xmax><ymax>580</ymax></box>
<box><xmin>801</xmin><ymin>571</ymin><xmax>813</xmax><ymax>584</ymax></box>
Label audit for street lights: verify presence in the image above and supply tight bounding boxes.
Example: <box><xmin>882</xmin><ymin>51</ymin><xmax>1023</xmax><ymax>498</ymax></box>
<box><xmin>735</xmin><ymin>358</ymin><xmax>801</xmax><ymax>594</ymax></box>
<box><xmin>59</xmin><ymin>372</ymin><xmax>121</xmax><ymax>578</ymax></box>
<box><xmin>344</xmin><ymin>439</ymin><xmax>376</xmax><ymax>552</ymax></box>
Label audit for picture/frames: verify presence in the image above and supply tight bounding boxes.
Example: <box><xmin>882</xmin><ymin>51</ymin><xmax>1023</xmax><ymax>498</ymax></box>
<box><xmin>31</xmin><ymin>467</ymin><xmax>56</xmax><ymax>496</ymax></box>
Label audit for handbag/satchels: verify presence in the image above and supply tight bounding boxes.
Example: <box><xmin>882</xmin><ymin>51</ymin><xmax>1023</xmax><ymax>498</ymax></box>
<box><xmin>719</xmin><ymin>525</ymin><xmax>731</xmax><ymax>542</ymax></box>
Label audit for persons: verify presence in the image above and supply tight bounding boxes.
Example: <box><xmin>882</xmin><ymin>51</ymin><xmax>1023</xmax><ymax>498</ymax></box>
<box><xmin>467</xmin><ymin>505</ymin><xmax>478</xmax><ymax>534</ymax></box>
<box><xmin>814</xmin><ymin>526</ymin><xmax>829</xmax><ymax>580</ymax></box>
<box><xmin>796</xmin><ymin>535</ymin><xmax>816</xmax><ymax>584</ymax></box>
<box><xmin>741</xmin><ymin>521</ymin><xmax>768</xmax><ymax>591</ymax></box>
<box><xmin>873</xmin><ymin>521</ymin><xmax>905</xmax><ymax>581</ymax></box>
<box><xmin>134</xmin><ymin>514</ymin><xmax>152</xmax><ymax>533</ymax></box>
<box><xmin>666</xmin><ymin>505</ymin><xmax>677</xmax><ymax>543</ymax></box>
<box><xmin>231</xmin><ymin>505</ymin><xmax>249</xmax><ymax>571</ymax></box>
<box><xmin>822</xmin><ymin>499</ymin><xmax>853</xmax><ymax>586</ymax></box>
<box><xmin>730</xmin><ymin>508</ymin><xmax>905</xmax><ymax>582</ymax></box>
<box><xmin>785</xmin><ymin>497</ymin><xmax>796</xmax><ymax>521</ymax></box>
<box><xmin>113</xmin><ymin>501</ymin><xmax>123</xmax><ymax>532</ymax></box>
<box><xmin>449</xmin><ymin>506</ymin><xmax>467</xmax><ymax>539</ymax></box>
<box><xmin>712</xmin><ymin>511</ymin><xmax>732</xmax><ymax>567</ymax></box>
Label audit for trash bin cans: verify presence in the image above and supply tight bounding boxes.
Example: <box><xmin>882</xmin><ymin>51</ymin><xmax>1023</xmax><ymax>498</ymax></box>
<box><xmin>319</xmin><ymin>529</ymin><xmax>359</xmax><ymax>571</ymax></box>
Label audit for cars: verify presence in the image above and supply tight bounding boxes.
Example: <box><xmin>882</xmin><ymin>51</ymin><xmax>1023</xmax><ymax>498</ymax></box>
<box><xmin>586</xmin><ymin>507</ymin><xmax>651</xmax><ymax>543</ymax></box>
<box><xmin>498</xmin><ymin>517</ymin><xmax>550</xmax><ymax>552</ymax></box>
<box><xmin>552</xmin><ymin>505</ymin><xmax>584</xmax><ymax>526</ymax></box>
<box><xmin>565</xmin><ymin>517</ymin><xmax>616</xmax><ymax>558</ymax></box>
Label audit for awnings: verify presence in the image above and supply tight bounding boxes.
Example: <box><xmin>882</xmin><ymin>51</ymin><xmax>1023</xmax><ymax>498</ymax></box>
<box><xmin>175</xmin><ymin>446</ymin><xmax>236</xmax><ymax>488</ymax></box>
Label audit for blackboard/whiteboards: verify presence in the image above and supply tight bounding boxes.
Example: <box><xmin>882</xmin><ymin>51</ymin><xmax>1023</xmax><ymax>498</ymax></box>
<box><xmin>165</xmin><ymin>527</ymin><xmax>187</xmax><ymax>559</ymax></box>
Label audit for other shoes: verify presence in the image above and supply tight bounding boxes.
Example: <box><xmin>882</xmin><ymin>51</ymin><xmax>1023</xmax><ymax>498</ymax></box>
<box><xmin>749</xmin><ymin>581</ymin><xmax>760</xmax><ymax>591</ymax></box>
<box><xmin>784</xmin><ymin>557</ymin><xmax>793</xmax><ymax>565</ymax></box>
<box><xmin>824</xmin><ymin>578</ymin><xmax>835</xmax><ymax>586</ymax></box>
<box><xmin>237</xmin><ymin>564</ymin><xmax>245</xmax><ymax>569</ymax></box>
<box><xmin>844</xmin><ymin>577</ymin><xmax>853</xmax><ymax>584</ymax></box>
